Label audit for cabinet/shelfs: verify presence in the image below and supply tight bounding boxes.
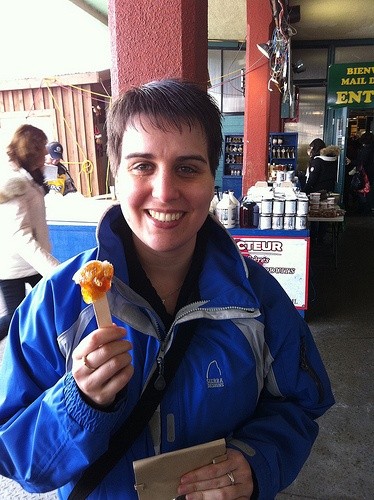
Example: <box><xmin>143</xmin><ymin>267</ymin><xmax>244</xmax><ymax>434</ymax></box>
<box><xmin>222</xmin><ymin>133</ymin><xmax>300</xmax><ymax>196</ymax></box>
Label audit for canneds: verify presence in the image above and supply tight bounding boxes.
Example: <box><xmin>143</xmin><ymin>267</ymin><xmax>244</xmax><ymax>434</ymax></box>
<box><xmin>276</xmin><ymin>171</ymin><xmax>294</xmax><ymax>183</ymax></box>
<box><xmin>259</xmin><ymin>198</ymin><xmax>309</xmax><ymax>230</ymax></box>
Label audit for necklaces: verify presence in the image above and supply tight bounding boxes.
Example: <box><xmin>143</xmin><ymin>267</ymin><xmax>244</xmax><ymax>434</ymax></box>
<box><xmin>161</xmin><ymin>286</ymin><xmax>181</xmax><ymax>304</ymax></box>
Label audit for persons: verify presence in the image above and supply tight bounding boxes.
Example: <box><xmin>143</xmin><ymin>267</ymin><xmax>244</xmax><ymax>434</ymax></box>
<box><xmin>0</xmin><ymin>125</ymin><xmax>62</xmax><ymax>340</ymax></box>
<box><xmin>301</xmin><ymin>133</ymin><xmax>374</xmax><ymax>247</ymax></box>
<box><xmin>45</xmin><ymin>141</ymin><xmax>77</xmax><ymax>196</ymax></box>
<box><xmin>0</xmin><ymin>77</ymin><xmax>335</xmax><ymax>500</ymax></box>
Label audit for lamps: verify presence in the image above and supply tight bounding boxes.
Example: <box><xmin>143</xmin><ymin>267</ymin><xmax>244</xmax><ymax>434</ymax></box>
<box><xmin>257</xmin><ymin>42</ymin><xmax>274</xmax><ymax>60</ymax></box>
<box><xmin>290</xmin><ymin>57</ymin><xmax>306</xmax><ymax>75</ymax></box>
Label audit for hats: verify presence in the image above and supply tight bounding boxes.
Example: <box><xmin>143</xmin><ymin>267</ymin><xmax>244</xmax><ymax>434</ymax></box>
<box><xmin>47</xmin><ymin>141</ymin><xmax>63</xmax><ymax>160</ymax></box>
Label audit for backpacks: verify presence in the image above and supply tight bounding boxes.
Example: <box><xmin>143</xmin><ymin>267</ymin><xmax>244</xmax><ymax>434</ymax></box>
<box><xmin>350</xmin><ymin>166</ymin><xmax>364</xmax><ymax>190</ymax></box>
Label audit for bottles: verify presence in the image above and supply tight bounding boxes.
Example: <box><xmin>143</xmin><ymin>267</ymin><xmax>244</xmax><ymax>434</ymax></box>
<box><xmin>207</xmin><ymin>189</ymin><xmax>240</xmax><ymax>228</ymax></box>
<box><xmin>226</xmin><ymin>144</ymin><xmax>242</xmax><ymax>163</ymax></box>
<box><xmin>272</xmin><ymin>136</ymin><xmax>296</xmax><ymax>159</ymax></box>
<box><xmin>239</xmin><ymin>201</ymin><xmax>260</xmax><ymax>228</ymax></box>
<box><xmin>270</xmin><ymin>162</ymin><xmax>294</xmax><ymax>181</ymax></box>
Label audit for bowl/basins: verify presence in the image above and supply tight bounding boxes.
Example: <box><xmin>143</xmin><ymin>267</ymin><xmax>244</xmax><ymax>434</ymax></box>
<box><xmin>309</xmin><ymin>192</ymin><xmax>335</xmax><ymax>209</ymax></box>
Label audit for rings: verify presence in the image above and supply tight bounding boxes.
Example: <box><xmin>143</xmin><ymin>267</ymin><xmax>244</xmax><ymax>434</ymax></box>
<box><xmin>82</xmin><ymin>355</ymin><xmax>95</xmax><ymax>371</ymax></box>
<box><xmin>227</xmin><ymin>472</ymin><xmax>234</xmax><ymax>485</ymax></box>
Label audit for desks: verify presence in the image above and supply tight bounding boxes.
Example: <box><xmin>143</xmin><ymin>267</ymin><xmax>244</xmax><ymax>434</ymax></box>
<box><xmin>308</xmin><ymin>202</ymin><xmax>345</xmax><ymax>268</ymax></box>
